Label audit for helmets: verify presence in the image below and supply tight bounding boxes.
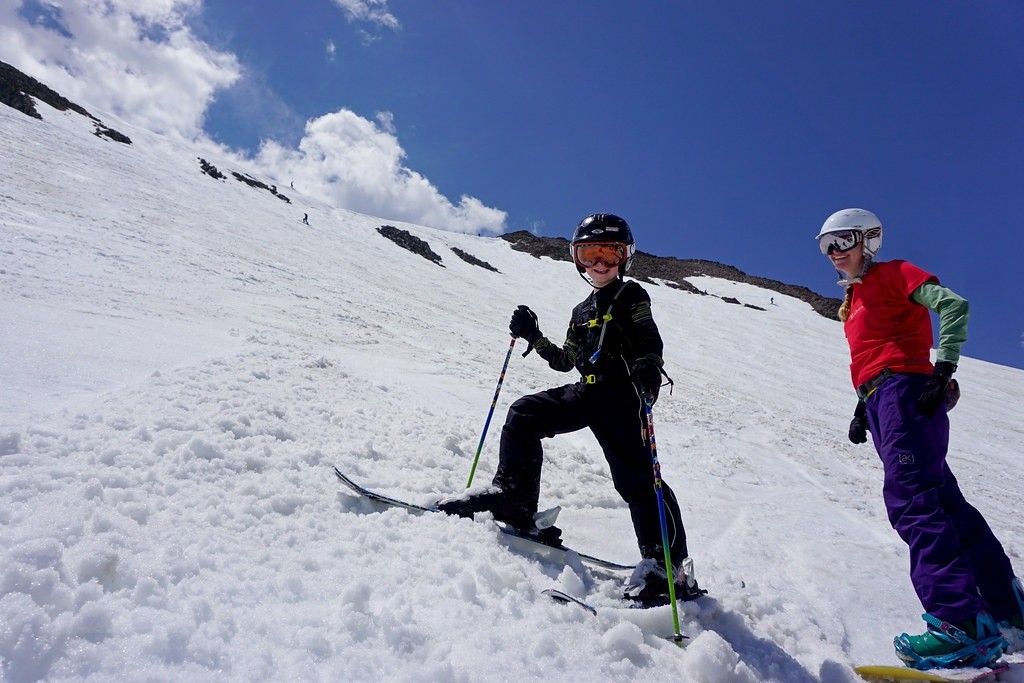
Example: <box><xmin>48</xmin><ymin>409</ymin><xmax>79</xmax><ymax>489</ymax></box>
<box><xmin>815</xmin><ymin>208</ymin><xmax>883</xmax><ymax>256</ymax></box>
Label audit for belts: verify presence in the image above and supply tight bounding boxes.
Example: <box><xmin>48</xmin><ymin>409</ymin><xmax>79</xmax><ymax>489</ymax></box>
<box><xmin>855</xmin><ymin>366</ymin><xmax>891</xmax><ymax>401</ymax></box>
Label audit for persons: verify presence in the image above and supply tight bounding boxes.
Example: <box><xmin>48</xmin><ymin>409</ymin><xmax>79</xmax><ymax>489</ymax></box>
<box><xmin>815</xmin><ymin>208</ymin><xmax>1024</xmax><ymax>669</ymax></box>
<box><xmin>303</xmin><ymin>213</ymin><xmax>308</xmax><ymax>225</ymax></box>
<box><xmin>438</xmin><ymin>213</ymin><xmax>698</xmax><ymax>603</ymax></box>
<box><xmin>291</xmin><ymin>181</ymin><xmax>293</xmax><ymax>188</ymax></box>
<box><xmin>771</xmin><ymin>298</ymin><xmax>773</xmax><ymax>304</ymax></box>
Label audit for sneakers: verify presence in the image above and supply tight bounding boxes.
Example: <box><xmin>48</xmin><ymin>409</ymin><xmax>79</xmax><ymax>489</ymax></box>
<box><xmin>982</xmin><ymin>578</ymin><xmax>1023</xmax><ymax>655</ymax></box>
<box><xmin>893</xmin><ymin>612</ymin><xmax>1009</xmax><ymax>672</ymax></box>
<box><xmin>436</xmin><ymin>481</ymin><xmax>539</xmax><ymax>530</ymax></box>
<box><xmin>620</xmin><ymin>555</ymin><xmax>699</xmax><ymax>604</ymax></box>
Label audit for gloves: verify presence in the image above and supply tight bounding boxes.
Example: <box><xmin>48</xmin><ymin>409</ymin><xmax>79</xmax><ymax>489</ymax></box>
<box><xmin>919</xmin><ymin>362</ymin><xmax>961</xmax><ymax>415</ymax></box>
<box><xmin>848</xmin><ymin>400</ymin><xmax>869</xmax><ymax>444</ymax></box>
<box><xmin>628</xmin><ymin>351</ymin><xmax>662</xmax><ymax>406</ymax></box>
<box><xmin>509</xmin><ymin>305</ymin><xmax>542</xmax><ymax>342</ymax></box>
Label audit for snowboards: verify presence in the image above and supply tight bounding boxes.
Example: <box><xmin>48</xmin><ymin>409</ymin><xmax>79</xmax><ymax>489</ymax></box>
<box><xmin>851</xmin><ymin>648</ymin><xmax>1024</xmax><ymax>683</ymax></box>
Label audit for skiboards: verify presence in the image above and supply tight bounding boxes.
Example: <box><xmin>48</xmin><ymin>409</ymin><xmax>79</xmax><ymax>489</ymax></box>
<box><xmin>331</xmin><ymin>463</ymin><xmax>644</xmax><ymax>572</ymax></box>
<box><xmin>538</xmin><ymin>578</ymin><xmax>747</xmax><ymax>617</ymax></box>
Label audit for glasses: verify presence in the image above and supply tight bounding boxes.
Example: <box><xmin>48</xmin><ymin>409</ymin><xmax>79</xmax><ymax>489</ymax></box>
<box><xmin>571</xmin><ymin>242</ymin><xmax>627</xmax><ymax>268</ymax></box>
<box><xmin>819</xmin><ymin>230</ymin><xmax>863</xmax><ymax>255</ymax></box>
<box><xmin>570</xmin><ymin>213</ymin><xmax>634</xmax><ymax>273</ymax></box>
